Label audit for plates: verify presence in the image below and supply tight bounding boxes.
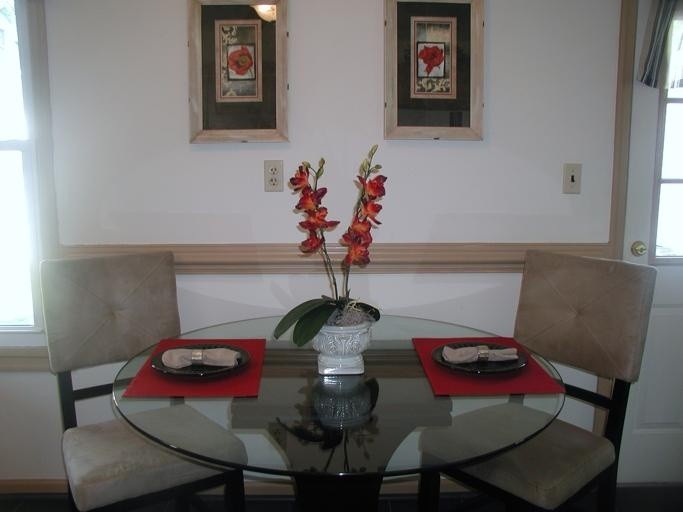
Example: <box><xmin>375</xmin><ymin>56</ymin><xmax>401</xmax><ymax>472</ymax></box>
<box><xmin>433</xmin><ymin>342</ymin><xmax>528</xmax><ymax>373</ymax></box>
<box><xmin>151</xmin><ymin>343</ymin><xmax>251</xmax><ymax>379</ymax></box>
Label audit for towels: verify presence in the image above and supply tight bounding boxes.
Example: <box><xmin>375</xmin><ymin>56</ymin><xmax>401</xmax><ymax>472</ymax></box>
<box><xmin>441</xmin><ymin>344</ymin><xmax>517</xmax><ymax>363</ymax></box>
<box><xmin>162</xmin><ymin>348</ymin><xmax>240</xmax><ymax>369</ymax></box>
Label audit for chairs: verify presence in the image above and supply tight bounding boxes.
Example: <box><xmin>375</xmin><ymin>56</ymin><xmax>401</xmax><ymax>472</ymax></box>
<box><xmin>39</xmin><ymin>251</ymin><xmax>246</xmax><ymax>512</ymax></box>
<box><xmin>418</xmin><ymin>250</ymin><xmax>658</xmax><ymax>512</ymax></box>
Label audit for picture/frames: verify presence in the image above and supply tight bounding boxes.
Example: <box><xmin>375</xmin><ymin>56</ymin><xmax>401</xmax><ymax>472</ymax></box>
<box><xmin>186</xmin><ymin>0</ymin><xmax>287</xmax><ymax>145</ymax></box>
<box><xmin>383</xmin><ymin>0</ymin><xmax>485</xmax><ymax>140</ymax></box>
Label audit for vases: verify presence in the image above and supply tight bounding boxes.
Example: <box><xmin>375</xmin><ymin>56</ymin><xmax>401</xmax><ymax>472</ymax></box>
<box><xmin>310</xmin><ymin>375</ymin><xmax>379</xmax><ymax>428</ymax></box>
<box><xmin>311</xmin><ymin>301</ymin><xmax>383</xmax><ymax>375</ymax></box>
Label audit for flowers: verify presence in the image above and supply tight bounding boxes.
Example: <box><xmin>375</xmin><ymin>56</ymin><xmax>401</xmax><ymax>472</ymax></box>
<box><xmin>273</xmin><ymin>144</ymin><xmax>386</xmax><ymax>346</ymax></box>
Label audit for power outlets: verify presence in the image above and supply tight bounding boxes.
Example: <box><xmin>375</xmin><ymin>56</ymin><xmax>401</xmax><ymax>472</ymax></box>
<box><xmin>264</xmin><ymin>160</ymin><xmax>283</xmax><ymax>193</ymax></box>
